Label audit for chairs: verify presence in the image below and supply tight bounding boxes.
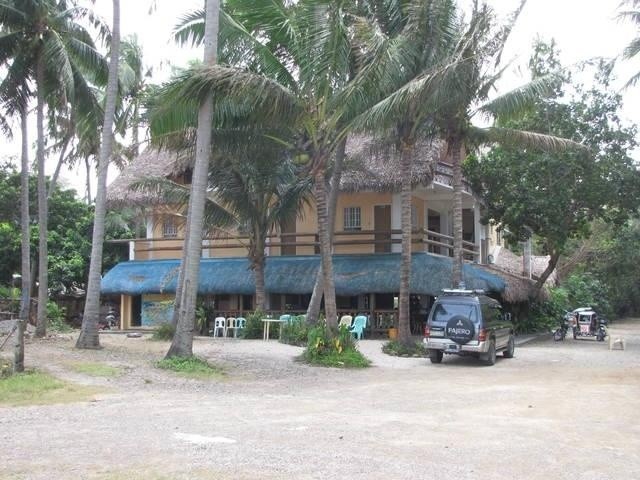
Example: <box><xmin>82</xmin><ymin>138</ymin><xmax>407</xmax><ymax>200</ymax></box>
<box><xmin>281</xmin><ymin>314</ymin><xmax>292</xmax><ymax>322</ymax></box>
<box><xmin>339</xmin><ymin>315</ymin><xmax>368</xmax><ymax>339</ymax></box>
<box><xmin>214</xmin><ymin>313</ymin><xmax>248</xmax><ymax>338</ymax></box>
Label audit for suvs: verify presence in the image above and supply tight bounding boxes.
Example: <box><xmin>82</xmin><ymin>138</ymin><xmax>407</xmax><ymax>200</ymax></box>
<box><xmin>423</xmin><ymin>288</ymin><xmax>515</xmax><ymax>365</ymax></box>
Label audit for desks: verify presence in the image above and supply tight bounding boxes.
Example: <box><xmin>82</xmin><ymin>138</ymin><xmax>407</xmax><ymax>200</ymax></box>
<box><xmin>262</xmin><ymin>318</ymin><xmax>286</xmax><ymax>341</ymax></box>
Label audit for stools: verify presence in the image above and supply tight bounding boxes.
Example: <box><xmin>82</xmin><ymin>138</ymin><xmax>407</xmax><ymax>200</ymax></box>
<box><xmin>607</xmin><ymin>334</ymin><xmax>625</xmax><ymax>352</ymax></box>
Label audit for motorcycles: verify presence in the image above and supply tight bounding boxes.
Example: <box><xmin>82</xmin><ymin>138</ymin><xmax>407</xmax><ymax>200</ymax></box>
<box><xmin>554</xmin><ymin>310</ymin><xmax>608</xmax><ymax>341</ymax></box>
